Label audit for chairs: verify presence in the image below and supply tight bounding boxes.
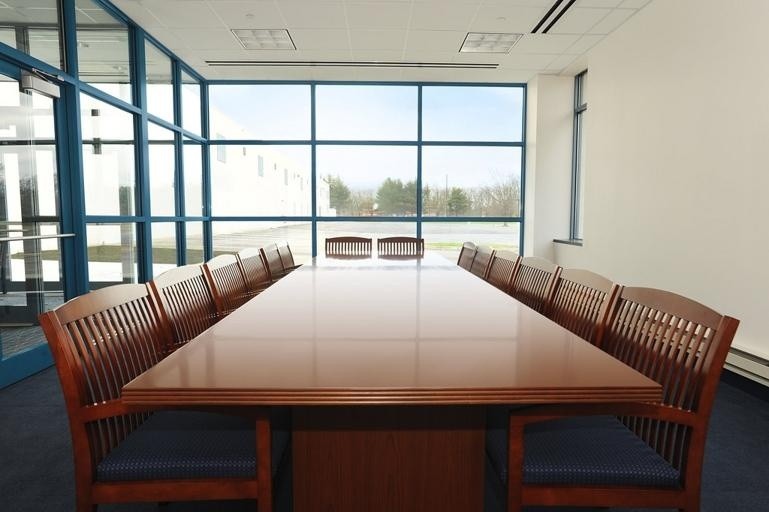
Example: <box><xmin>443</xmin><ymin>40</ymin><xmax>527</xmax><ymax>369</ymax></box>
<box><xmin>324</xmin><ymin>234</ymin><xmax>425</xmax><ymax>257</ymax></box>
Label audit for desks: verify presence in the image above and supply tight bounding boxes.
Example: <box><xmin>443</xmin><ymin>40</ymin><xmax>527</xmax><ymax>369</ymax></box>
<box><xmin>121</xmin><ymin>254</ymin><xmax>663</xmax><ymax>511</ymax></box>
